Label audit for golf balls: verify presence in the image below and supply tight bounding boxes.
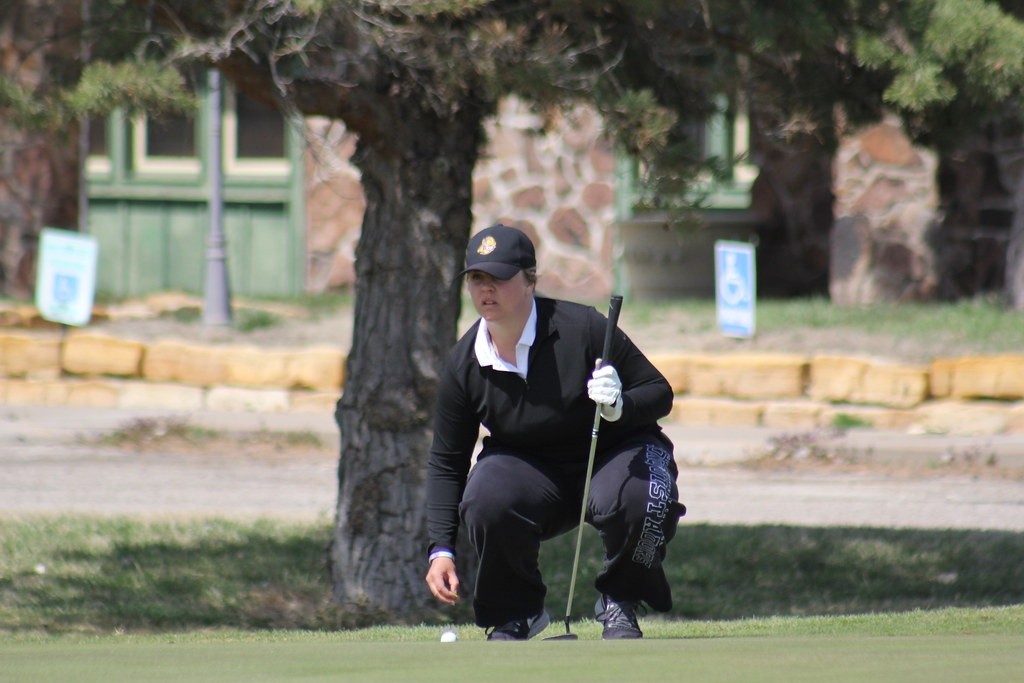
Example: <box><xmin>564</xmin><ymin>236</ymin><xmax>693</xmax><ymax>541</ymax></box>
<box><xmin>440</xmin><ymin>631</ymin><xmax>457</xmax><ymax>641</ymax></box>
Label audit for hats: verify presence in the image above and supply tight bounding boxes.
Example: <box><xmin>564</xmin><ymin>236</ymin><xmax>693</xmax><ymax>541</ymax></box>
<box><xmin>453</xmin><ymin>223</ymin><xmax>537</xmax><ymax>284</ymax></box>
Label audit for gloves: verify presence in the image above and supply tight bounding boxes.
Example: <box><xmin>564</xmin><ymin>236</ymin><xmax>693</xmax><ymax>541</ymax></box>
<box><xmin>587</xmin><ymin>359</ymin><xmax>622</xmax><ymax>413</ymax></box>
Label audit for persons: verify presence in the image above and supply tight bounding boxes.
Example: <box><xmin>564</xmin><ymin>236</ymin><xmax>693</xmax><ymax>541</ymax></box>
<box><xmin>425</xmin><ymin>223</ymin><xmax>688</xmax><ymax>641</ymax></box>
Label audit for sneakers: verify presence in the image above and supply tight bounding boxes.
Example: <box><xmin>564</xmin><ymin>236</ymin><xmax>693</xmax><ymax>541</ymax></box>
<box><xmin>593</xmin><ymin>592</ymin><xmax>643</xmax><ymax>641</ymax></box>
<box><xmin>488</xmin><ymin>606</ymin><xmax>552</xmax><ymax>642</ymax></box>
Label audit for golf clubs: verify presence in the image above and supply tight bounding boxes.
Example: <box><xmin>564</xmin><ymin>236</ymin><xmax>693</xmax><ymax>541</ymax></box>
<box><xmin>543</xmin><ymin>294</ymin><xmax>623</xmax><ymax>643</ymax></box>
